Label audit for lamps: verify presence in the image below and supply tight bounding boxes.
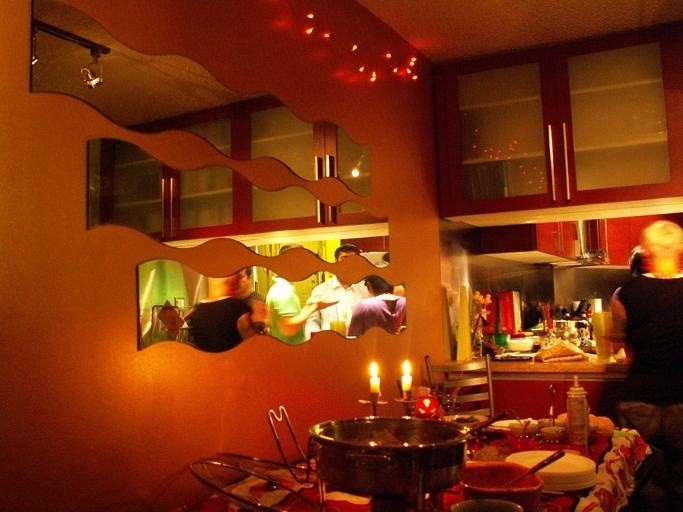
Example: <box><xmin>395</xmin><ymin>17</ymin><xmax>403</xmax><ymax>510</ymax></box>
<box><xmin>32</xmin><ymin>19</ymin><xmax>111</xmax><ymax>88</ymax></box>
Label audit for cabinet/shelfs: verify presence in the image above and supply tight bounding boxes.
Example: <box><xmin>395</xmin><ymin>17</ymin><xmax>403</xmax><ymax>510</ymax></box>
<box><xmin>477</xmin><ymin>221</ymin><xmax>576</xmax><ymax>267</ymax></box>
<box><xmin>583</xmin><ymin>214</ymin><xmax>649</xmax><ymax>270</ymax></box>
<box><xmin>249</xmin><ymin>107</ymin><xmax>369</xmax><ymax>224</ymax></box>
<box><xmin>436</xmin><ymin>30</ymin><xmax>683</xmax><ymax>227</ymax></box>
<box><xmin>111</xmin><ymin>116</ymin><xmax>238</xmax><ymax>240</ymax></box>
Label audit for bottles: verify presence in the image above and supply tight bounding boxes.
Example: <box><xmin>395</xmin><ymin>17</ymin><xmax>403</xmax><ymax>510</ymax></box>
<box><xmin>562</xmin><ymin>375</ymin><xmax>591</xmax><ymax>455</ymax></box>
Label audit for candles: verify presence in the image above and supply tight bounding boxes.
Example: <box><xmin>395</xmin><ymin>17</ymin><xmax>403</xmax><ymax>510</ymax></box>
<box><xmin>368</xmin><ymin>362</ymin><xmax>380</xmax><ymax>397</ymax></box>
<box><xmin>400</xmin><ymin>359</ymin><xmax>413</xmax><ymax>391</ymax></box>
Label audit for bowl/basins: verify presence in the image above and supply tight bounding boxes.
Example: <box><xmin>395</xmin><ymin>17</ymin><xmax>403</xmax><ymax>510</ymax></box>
<box><xmin>459</xmin><ymin>457</ymin><xmax>542</xmax><ymax>507</ymax></box>
<box><xmin>448</xmin><ymin>498</ymin><xmax>524</xmax><ymax>511</ymax></box>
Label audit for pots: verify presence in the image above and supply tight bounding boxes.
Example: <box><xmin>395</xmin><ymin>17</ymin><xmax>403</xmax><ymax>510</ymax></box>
<box><xmin>264</xmin><ymin>404</ymin><xmax>470</xmax><ymax>510</ymax></box>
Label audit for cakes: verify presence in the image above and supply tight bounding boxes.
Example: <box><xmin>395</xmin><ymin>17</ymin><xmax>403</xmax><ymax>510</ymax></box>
<box><xmin>510</xmin><ymin>412</ymin><xmax>615</xmax><ymax>443</ymax></box>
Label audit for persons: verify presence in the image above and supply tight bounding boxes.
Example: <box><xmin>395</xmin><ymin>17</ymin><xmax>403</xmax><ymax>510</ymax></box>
<box><xmin>187</xmin><ymin>242</ymin><xmax>415</xmax><ymax>352</ymax></box>
<box><xmin>609</xmin><ymin>222</ymin><xmax>683</xmax><ymax>439</ymax></box>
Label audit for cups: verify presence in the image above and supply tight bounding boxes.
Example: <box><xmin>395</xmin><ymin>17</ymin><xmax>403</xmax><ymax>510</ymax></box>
<box><xmin>571</xmin><ymin>296</ymin><xmax>614</xmax><ymax>360</ymax></box>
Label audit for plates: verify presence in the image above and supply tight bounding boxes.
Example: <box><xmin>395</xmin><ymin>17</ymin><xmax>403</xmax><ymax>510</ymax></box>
<box><xmin>504</xmin><ymin>449</ymin><xmax>597</xmax><ymax>492</ymax></box>
<box><xmin>440</xmin><ymin>414</ymin><xmax>487</xmax><ymax>427</ymax></box>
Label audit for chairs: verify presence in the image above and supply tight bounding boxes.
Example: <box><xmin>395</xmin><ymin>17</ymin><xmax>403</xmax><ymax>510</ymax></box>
<box><xmin>422</xmin><ymin>352</ymin><xmax>493</xmax><ymax>420</ymax></box>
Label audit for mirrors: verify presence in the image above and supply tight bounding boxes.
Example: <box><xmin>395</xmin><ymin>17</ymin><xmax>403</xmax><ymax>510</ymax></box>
<box><xmin>134</xmin><ymin>258</ymin><xmax>405</xmax><ymax>352</ymax></box>
<box><xmin>29</xmin><ymin>0</ymin><xmax>371</xmax><ymax>201</ymax></box>
<box><xmin>85</xmin><ymin>137</ymin><xmax>389</xmax><ymax>269</ymax></box>
<box><xmin>434</xmin><ymin>210</ymin><xmax>682</xmax><ymax>361</ymax></box>
